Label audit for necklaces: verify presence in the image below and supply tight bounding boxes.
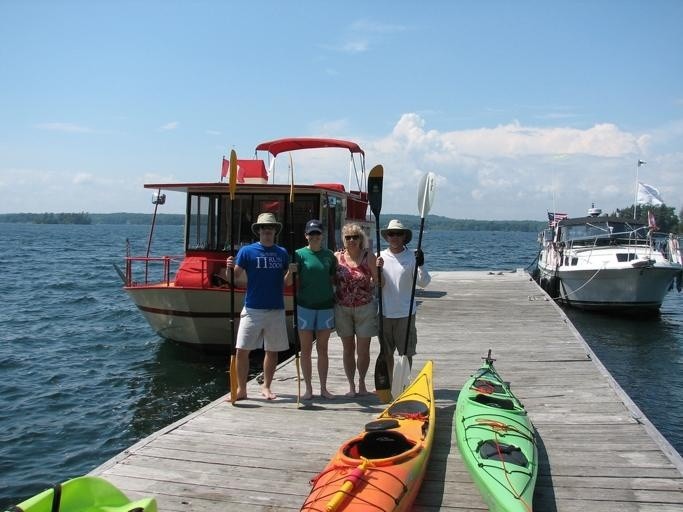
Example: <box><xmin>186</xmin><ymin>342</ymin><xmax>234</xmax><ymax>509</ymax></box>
<box><xmin>348</xmin><ymin>252</ymin><xmax>363</xmax><ymax>266</ymax></box>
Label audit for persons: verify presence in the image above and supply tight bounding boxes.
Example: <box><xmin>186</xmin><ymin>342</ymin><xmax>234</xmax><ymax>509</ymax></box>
<box><xmin>332</xmin><ymin>224</ymin><xmax>385</xmax><ymax>399</ymax></box>
<box><xmin>283</xmin><ymin>220</ymin><xmax>337</xmax><ymax>401</ymax></box>
<box><xmin>374</xmin><ymin>220</ymin><xmax>432</xmax><ymax>394</ymax></box>
<box><xmin>667</xmin><ymin>233</ymin><xmax>683</xmax><ymax>266</ymax></box>
<box><xmin>225</xmin><ymin>212</ymin><xmax>289</xmax><ymax>401</ymax></box>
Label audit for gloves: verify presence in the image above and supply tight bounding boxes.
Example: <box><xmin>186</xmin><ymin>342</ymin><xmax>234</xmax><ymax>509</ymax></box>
<box><xmin>414</xmin><ymin>250</ymin><xmax>424</xmax><ymax>266</ymax></box>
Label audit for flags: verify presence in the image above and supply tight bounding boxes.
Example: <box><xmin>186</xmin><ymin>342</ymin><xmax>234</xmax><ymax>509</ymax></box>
<box><xmin>549</xmin><ymin>212</ymin><xmax>568</xmax><ymax>227</ymax></box>
<box><xmin>637</xmin><ymin>182</ymin><xmax>664</xmax><ymax>207</ymax></box>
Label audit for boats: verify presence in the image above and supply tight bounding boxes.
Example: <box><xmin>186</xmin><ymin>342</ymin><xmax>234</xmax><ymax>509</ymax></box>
<box><xmin>297</xmin><ymin>356</ymin><xmax>436</xmax><ymax>512</ymax></box>
<box><xmin>4</xmin><ymin>476</ymin><xmax>157</xmax><ymax>512</ymax></box>
<box><xmin>532</xmin><ymin>156</ymin><xmax>682</xmax><ymax>311</ymax></box>
<box><xmin>111</xmin><ymin>137</ymin><xmax>376</xmax><ymax>346</ymax></box>
<box><xmin>453</xmin><ymin>348</ymin><xmax>542</xmax><ymax>512</ymax></box>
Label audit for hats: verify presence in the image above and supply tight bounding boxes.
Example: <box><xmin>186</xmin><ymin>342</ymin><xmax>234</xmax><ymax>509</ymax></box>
<box><xmin>381</xmin><ymin>219</ymin><xmax>411</xmax><ymax>243</ymax></box>
<box><xmin>251</xmin><ymin>212</ymin><xmax>282</xmax><ymax>237</ymax></box>
<box><xmin>305</xmin><ymin>220</ymin><xmax>323</xmax><ymax>234</ymax></box>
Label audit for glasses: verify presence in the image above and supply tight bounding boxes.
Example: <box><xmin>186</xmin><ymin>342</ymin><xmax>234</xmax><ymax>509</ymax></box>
<box><xmin>387</xmin><ymin>231</ymin><xmax>404</xmax><ymax>237</ymax></box>
<box><xmin>345</xmin><ymin>235</ymin><xmax>359</xmax><ymax>240</ymax></box>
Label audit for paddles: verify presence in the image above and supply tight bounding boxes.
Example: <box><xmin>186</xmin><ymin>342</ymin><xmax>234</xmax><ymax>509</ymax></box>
<box><xmin>287</xmin><ymin>152</ymin><xmax>301</xmax><ymax>405</ymax></box>
<box><xmin>368</xmin><ymin>164</ymin><xmax>393</xmax><ymax>404</ymax></box>
<box><xmin>393</xmin><ymin>172</ymin><xmax>435</xmax><ymax>402</ymax></box>
<box><xmin>229</xmin><ymin>150</ymin><xmax>237</xmax><ymax>406</ymax></box>
